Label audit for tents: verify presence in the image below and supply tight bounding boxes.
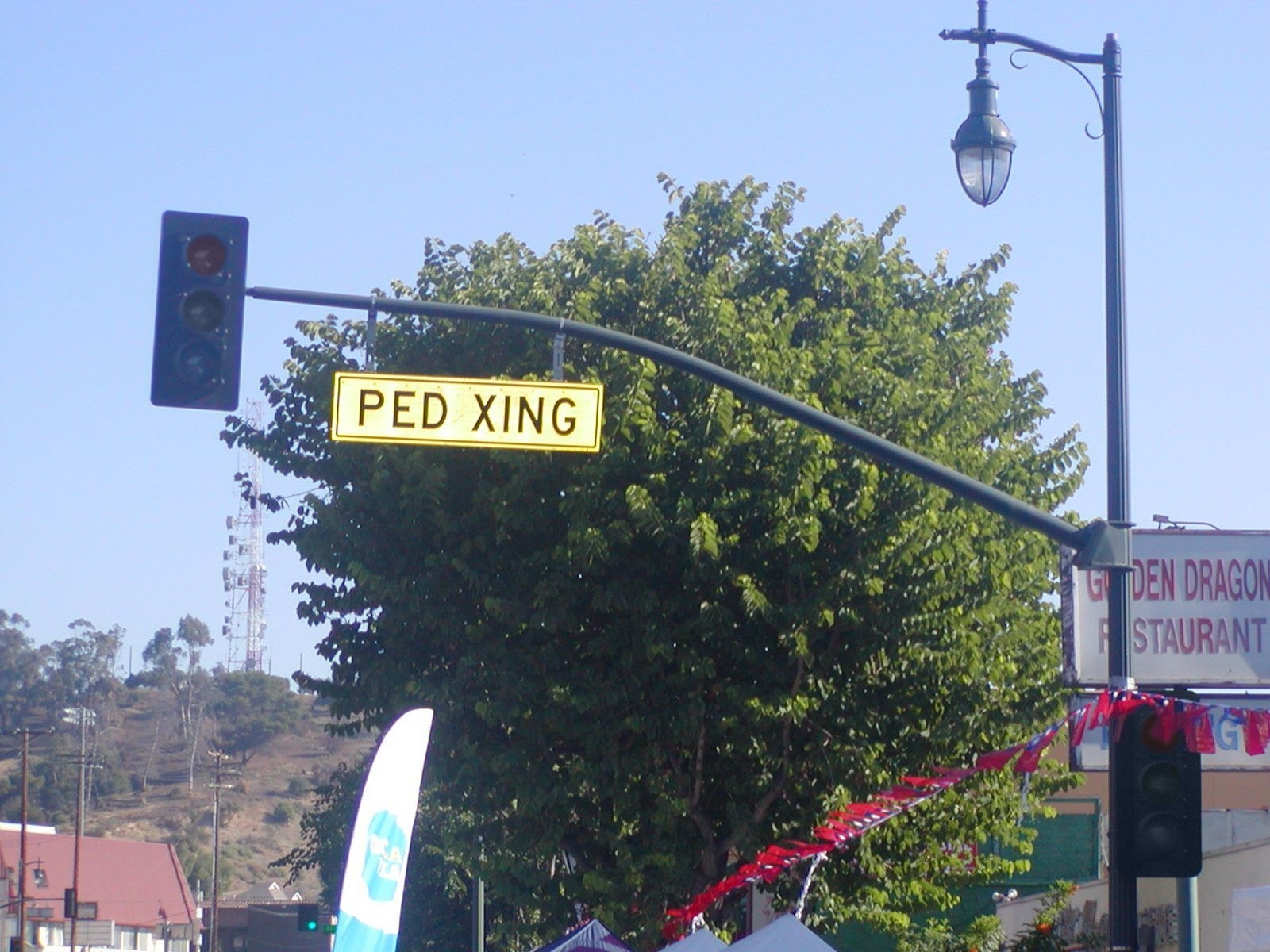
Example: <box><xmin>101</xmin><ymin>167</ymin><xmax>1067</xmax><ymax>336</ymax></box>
<box><xmin>531</xmin><ymin>913</ymin><xmax>836</xmax><ymax>952</ymax></box>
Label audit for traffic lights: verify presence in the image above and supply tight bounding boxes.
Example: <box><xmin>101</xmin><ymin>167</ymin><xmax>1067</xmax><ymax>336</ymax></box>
<box><xmin>298</xmin><ymin>903</ymin><xmax>319</xmax><ymax>933</ymax></box>
<box><xmin>151</xmin><ymin>209</ymin><xmax>250</xmax><ymax>411</ymax></box>
<box><xmin>1110</xmin><ymin>696</ymin><xmax>1203</xmax><ymax>879</ymax></box>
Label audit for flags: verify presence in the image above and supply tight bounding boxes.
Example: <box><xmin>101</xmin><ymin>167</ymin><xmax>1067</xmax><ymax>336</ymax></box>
<box><xmin>333</xmin><ymin>708</ymin><xmax>434</xmax><ymax>952</ymax></box>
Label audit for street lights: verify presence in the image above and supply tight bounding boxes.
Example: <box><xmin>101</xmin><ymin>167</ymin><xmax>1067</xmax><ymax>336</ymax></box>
<box><xmin>939</xmin><ymin>0</ymin><xmax>1139</xmax><ymax>952</ymax></box>
